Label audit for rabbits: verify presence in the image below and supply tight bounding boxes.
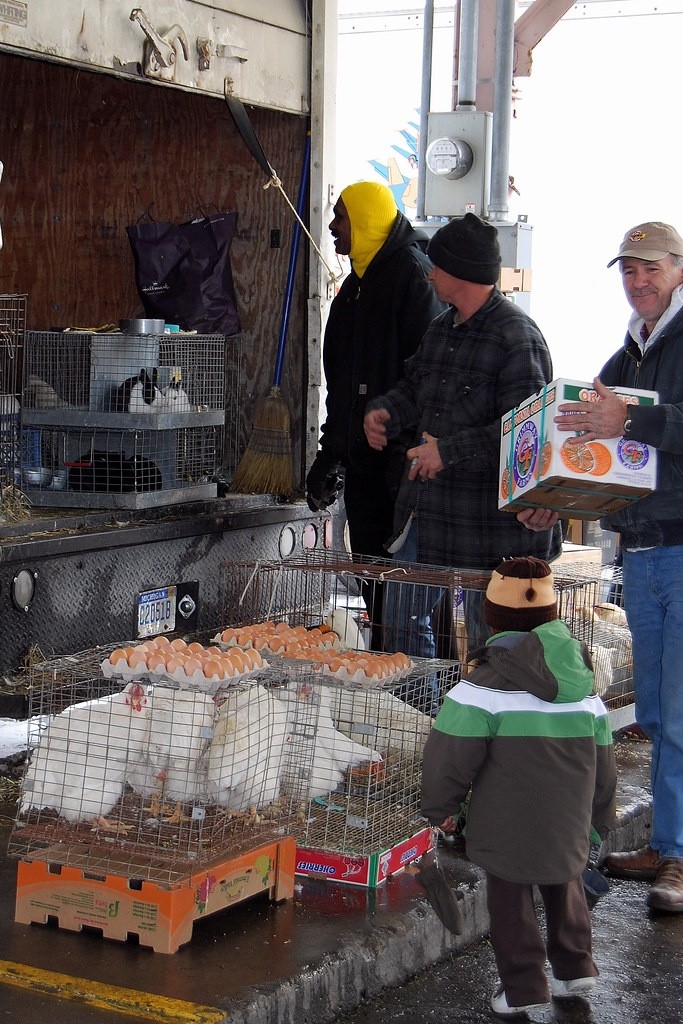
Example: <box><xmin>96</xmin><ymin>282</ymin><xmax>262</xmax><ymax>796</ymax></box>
<box><xmin>68</xmin><ymin>449</ymin><xmax>162</xmax><ymax>494</ymax></box>
<box><xmin>115</xmin><ymin>367</ymin><xmax>191</xmax><ymax>415</ymax></box>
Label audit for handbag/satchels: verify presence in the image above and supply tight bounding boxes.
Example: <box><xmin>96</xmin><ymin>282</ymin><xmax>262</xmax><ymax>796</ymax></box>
<box><xmin>125</xmin><ymin>203</ymin><xmax>243</xmax><ymax>338</ymax></box>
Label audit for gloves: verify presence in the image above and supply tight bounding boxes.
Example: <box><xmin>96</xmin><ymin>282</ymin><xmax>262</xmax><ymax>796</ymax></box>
<box><xmin>415</xmin><ymin>859</ymin><xmax>464</xmax><ymax>936</ymax></box>
<box><xmin>307</xmin><ymin>449</ymin><xmax>343</xmax><ymax>513</ymax></box>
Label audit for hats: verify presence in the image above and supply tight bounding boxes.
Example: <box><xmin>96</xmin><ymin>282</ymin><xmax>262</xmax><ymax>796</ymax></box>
<box><xmin>606</xmin><ymin>222</ymin><xmax>683</xmax><ymax>271</ymax></box>
<box><xmin>427</xmin><ymin>212</ymin><xmax>502</xmax><ymax>286</ymax></box>
<box><xmin>484</xmin><ymin>556</ymin><xmax>557</xmax><ymax>631</ymax></box>
<box><xmin>341</xmin><ymin>179</ymin><xmax>398</xmax><ymax>279</ymax></box>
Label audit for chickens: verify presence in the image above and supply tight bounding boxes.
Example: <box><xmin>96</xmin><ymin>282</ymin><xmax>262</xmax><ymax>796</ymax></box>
<box><xmin>24</xmin><ymin>374</ymin><xmax>91</xmax><ymax>471</ymax></box>
<box><xmin>575</xmin><ymin>603</ymin><xmax>632</xmax><ymax>697</ymax></box>
<box><xmin>15</xmin><ymin>679</ymin><xmax>384</xmax><ymax>835</ymax></box>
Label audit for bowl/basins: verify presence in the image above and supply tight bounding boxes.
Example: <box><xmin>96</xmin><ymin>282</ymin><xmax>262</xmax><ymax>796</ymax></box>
<box><xmin>23</xmin><ymin>466</ymin><xmax>53</xmax><ymax>487</ymax></box>
<box><xmin>49</xmin><ymin>470</ymin><xmax>69</xmax><ymax>490</ymax></box>
<box><xmin>119</xmin><ymin>317</ymin><xmax>165</xmax><ymax>335</ymax></box>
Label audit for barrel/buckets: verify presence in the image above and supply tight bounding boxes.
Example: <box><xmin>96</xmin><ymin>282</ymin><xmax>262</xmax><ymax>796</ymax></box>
<box><xmin>582</xmin><ymin>866</ymin><xmax>609</xmax><ymax>911</ymax></box>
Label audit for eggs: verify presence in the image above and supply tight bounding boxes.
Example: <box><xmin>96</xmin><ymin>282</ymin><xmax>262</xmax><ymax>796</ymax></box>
<box><xmin>108</xmin><ymin>621</ymin><xmax>410</xmax><ymax>678</ymax></box>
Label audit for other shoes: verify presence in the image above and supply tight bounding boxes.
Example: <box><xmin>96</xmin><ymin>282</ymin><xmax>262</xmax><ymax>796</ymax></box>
<box><xmin>552</xmin><ymin>972</ymin><xmax>595</xmax><ymax>998</ymax></box>
<box><xmin>490</xmin><ymin>984</ymin><xmax>551</xmax><ymax>1014</ymax></box>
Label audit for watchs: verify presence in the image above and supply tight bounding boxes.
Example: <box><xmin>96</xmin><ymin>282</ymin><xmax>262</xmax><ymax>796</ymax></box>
<box><xmin>624</xmin><ymin>405</ymin><xmax>632</xmax><ymax>436</ymax></box>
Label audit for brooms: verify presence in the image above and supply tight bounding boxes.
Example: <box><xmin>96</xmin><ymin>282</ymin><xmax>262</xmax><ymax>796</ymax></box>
<box><xmin>227</xmin><ymin>122</ymin><xmax>314</xmax><ymax>496</ymax></box>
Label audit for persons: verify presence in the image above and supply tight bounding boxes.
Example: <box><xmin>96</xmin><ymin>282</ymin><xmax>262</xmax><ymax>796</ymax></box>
<box><xmin>519</xmin><ymin>221</ymin><xmax>683</xmax><ymax>910</ymax></box>
<box><xmin>362</xmin><ymin>211</ymin><xmax>556</xmax><ymax>717</ymax></box>
<box><xmin>420</xmin><ymin>554</ymin><xmax>618</xmax><ymax>1012</ymax></box>
<box><xmin>306</xmin><ymin>181</ymin><xmax>461</xmax><ymax>717</ymax></box>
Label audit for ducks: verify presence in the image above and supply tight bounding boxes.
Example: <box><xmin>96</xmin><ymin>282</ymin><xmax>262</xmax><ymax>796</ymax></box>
<box><xmin>320</xmin><ymin>609</ymin><xmax>436</xmax><ymax>781</ymax></box>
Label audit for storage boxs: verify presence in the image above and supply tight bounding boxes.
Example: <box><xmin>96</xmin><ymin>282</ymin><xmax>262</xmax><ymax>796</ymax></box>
<box><xmin>0</xmin><ymin>291</ymin><xmax>660</xmax><ymax>953</ymax></box>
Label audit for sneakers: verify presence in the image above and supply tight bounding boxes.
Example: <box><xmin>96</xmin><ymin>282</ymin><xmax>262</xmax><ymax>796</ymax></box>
<box><xmin>604</xmin><ymin>842</ymin><xmax>663</xmax><ymax>878</ymax></box>
<box><xmin>644</xmin><ymin>858</ymin><xmax>683</xmax><ymax>913</ymax></box>
